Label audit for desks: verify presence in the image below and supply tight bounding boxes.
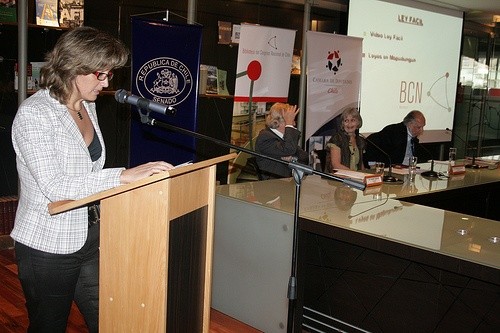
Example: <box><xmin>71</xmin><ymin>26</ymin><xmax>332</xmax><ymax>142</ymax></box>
<box><xmin>210</xmin><ymin>155</ymin><xmax>500</xmax><ymax>333</ymax></box>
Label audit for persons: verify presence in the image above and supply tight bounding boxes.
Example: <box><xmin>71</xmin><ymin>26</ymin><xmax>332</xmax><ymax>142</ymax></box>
<box><xmin>369</xmin><ymin>110</ymin><xmax>426</xmax><ymax>166</ymax></box>
<box><xmin>10</xmin><ymin>27</ymin><xmax>175</xmax><ymax>333</ymax></box>
<box><xmin>325</xmin><ymin>108</ymin><xmax>367</xmax><ymax>166</ymax></box>
<box><xmin>255</xmin><ymin>102</ymin><xmax>309</xmax><ymax>181</ymax></box>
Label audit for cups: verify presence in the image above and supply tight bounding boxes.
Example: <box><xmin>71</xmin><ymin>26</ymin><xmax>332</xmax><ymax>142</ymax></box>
<box><xmin>375</xmin><ymin>163</ymin><xmax>384</xmax><ymax>177</ymax></box>
<box><xmin>409</xmin><ymin>156</ymin><xmax>417</xmax><ymax>174</ymax></box>
<box><xmin>448</xmin><ymin>148</ymin><xmax>456</xmax><ymax>162</ymax></box>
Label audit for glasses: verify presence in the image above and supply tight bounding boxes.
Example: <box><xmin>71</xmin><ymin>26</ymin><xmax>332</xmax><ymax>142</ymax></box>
<box><xmin>92</xmin><ymin>70</ymin><xmax>114</xmax><ymax>82</ymax></box>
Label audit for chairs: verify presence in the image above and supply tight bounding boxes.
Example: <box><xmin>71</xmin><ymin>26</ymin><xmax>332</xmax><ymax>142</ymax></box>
<box><xmin>313</xmin><ymin>149</ymin><xmax>327</xmax><ymax>172</ymax></box>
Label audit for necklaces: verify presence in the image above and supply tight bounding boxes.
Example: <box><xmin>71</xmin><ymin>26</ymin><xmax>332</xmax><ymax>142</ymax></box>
<box><xmin>66</xmin><ymin>103</ymin><xmax>83</xmax><ymax>120</ymax></box>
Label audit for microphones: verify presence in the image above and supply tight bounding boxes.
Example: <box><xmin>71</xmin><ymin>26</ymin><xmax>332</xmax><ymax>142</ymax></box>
<box><xmin>355</xmin><ymin>132</ymin><xmax>396</xmax><ymax>182</ymax></box>
<box><xmin>446</xmin><ymin>128</ymin><xmax>480</xmax><ymax>169</ymax></box>
<box><xmin>402</xmin><ymin>130</ymin><xmax>437</xmax><ymax>178</ymax></box>
<box><xmin>114</xmin><ymin>88</ymin><xmax>169</xmax><ymax>115</ymax></box>
